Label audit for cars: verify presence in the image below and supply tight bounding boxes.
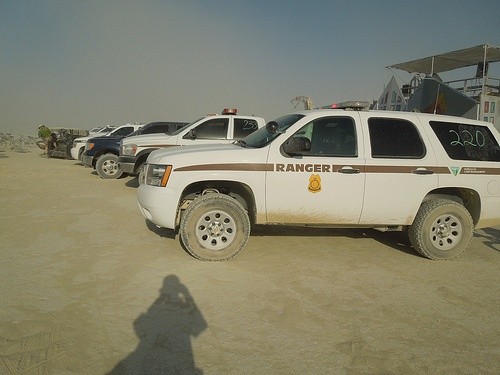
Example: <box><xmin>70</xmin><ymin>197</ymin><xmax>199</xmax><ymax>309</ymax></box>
<box><xmin>70</xmin><ymin>124</ymin><xmax>191</xmax><ymax>180</ymax></box>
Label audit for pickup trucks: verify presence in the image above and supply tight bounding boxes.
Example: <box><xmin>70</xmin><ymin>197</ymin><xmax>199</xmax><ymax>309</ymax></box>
<box><xmin>122</xmin><ymin>109</ymin><xmax>272</xmax><ymax>179</ymax></box>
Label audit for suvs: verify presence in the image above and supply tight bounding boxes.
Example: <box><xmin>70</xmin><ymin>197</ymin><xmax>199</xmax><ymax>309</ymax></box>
<box><xmin>136</xmin><ymin>100</ymin><xmax>499</xmax><ymax>259</ymax></box>
